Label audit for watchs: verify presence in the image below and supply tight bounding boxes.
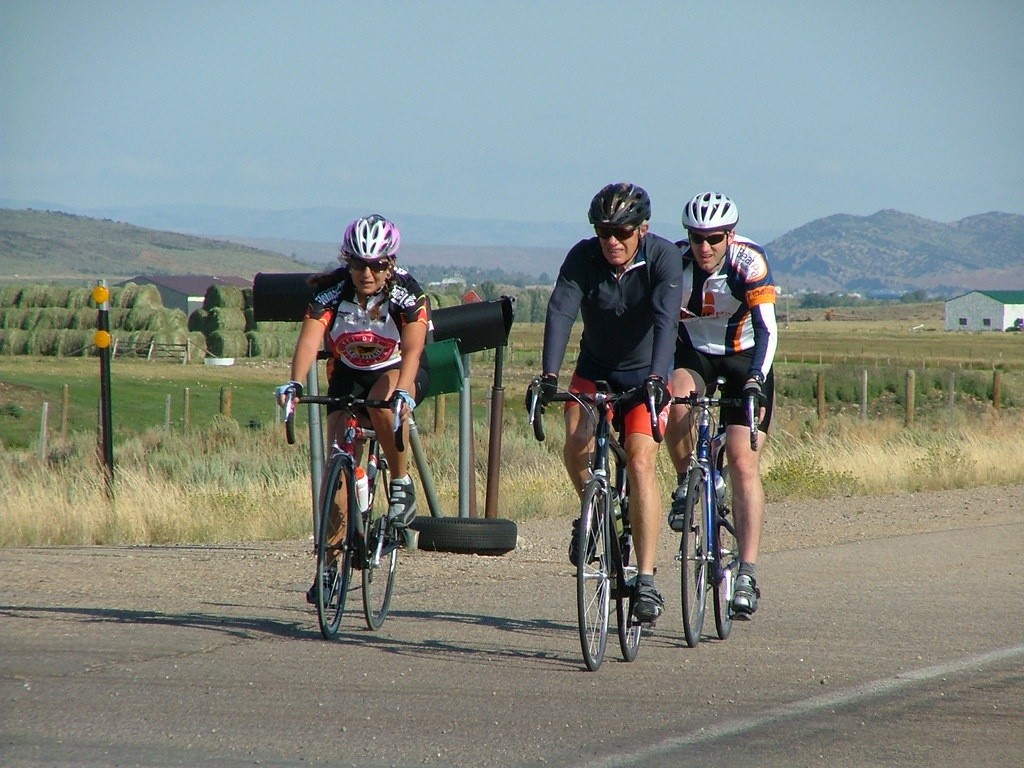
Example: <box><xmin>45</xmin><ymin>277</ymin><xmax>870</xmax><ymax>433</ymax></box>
<box><xmin>745</xmin><ymin>375</ymin><xmax>763</xmax><ymax>390</ymax></box>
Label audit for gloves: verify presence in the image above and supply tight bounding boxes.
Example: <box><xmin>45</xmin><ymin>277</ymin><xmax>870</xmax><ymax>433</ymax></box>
<box><xmin>643</xmin><ymin>378</ymin><xmax>672</xmax><ymax>416</ymax></box>
<box><xmin>525</xmin><ymin>375</ymin><xmax>558</xmax><ymax>414</ymax></box>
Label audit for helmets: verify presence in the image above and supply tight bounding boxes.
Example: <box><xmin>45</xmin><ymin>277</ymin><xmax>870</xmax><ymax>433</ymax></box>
<box><xmin>341</xmin><ymin>214</ymin><xmax>399</xmax><ymax>260</ymax></box>
<box><xmin>682</xmin><ymin>191</ymin><xmax>739</xmax><ymax>232</ymax></box>
<box><xmin>588</xmin><ymin>184</ymin><xmax>651</xmax><ymax>226</ymax></box>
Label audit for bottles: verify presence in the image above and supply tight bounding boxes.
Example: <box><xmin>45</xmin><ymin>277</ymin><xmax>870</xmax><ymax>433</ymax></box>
<box><xmin>712</xmin><ymin>469</ymin><xmax>730</xmax><ymax>505</ymax></box>
<box><xmin>608</xmin><ymin>485</ymin><xmax>625</xmax><ymax>539</ymax></box>
<box><xmin>354</xmin><ymin>467</ymin><xmax>370</xmax><ymax>513</ymax></box>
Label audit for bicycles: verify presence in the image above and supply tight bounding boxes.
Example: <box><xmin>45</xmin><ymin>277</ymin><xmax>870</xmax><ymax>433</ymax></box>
<box><xmin>663</xmin><ymin>377</ymin><xmax>752</xmax><ymax>649</ymax></box>
<box><xmin>284</xmin><ymin>386</ymin><xmax>413</xmax><ymax>641</ymax></box>
<box><xmin>529</xmin><ymin>378</ymin><xmax>662</xmax><ymax>672</ymax></box>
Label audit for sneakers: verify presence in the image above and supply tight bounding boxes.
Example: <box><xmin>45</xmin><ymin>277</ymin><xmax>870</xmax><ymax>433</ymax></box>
<box><xmin>306</xmin><ymin>565</ymin><xmax>342</xmax><ymax>603</ymax></box>
<box><xmin>632</xmin><ymin>580</ymin><xmax>665</xmax><ymax>621</ymax></box>
<box><xmin>568</xmin><ymin>516</ymin><xmax>602</xmax><ymax>566</ymax></box>
<box><xmin>730</xmin><ymin>563</ymin><xmax>761</xmax><ymax>614</ymax></box>
<box><xmin>387</xmin><ymin>472</ymin><xmax>418</xmax><ymax>527</ymax></box>
<box><xmin>669</xmin><ymin>478</ymin><xmax>699</xmax><ymax>531</ymax></box>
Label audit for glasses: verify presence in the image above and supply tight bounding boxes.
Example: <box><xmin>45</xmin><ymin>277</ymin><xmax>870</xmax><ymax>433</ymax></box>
<box><xmin>346</xmin><ymin>254</ymin><xmax>392</xmax><ymax>273</ymax></box>
<box><xmin>688</xmin><ymin>228</ymin><xmax>728</xmax><ymax>245</ymax></box>
<box><xmin>594</xmin><ymin>221</ymin><xmax>645</xmax><ymax>241</ymax></box>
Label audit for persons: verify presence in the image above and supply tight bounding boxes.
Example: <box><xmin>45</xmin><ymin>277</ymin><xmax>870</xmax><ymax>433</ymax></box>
<box><xmin>276</xmin><ymin>214</ymin><xmax>427</xmax><ymax>602</ymax></box>
<box><xmin>668</xmin><ymin>191</ymin><xmax>778</xmax><ymax>613</ymax></box>
<box><xmin>526</xmin><ymin>183</ymin><xmax>683</xmax><ymax>621</ymax></box>
